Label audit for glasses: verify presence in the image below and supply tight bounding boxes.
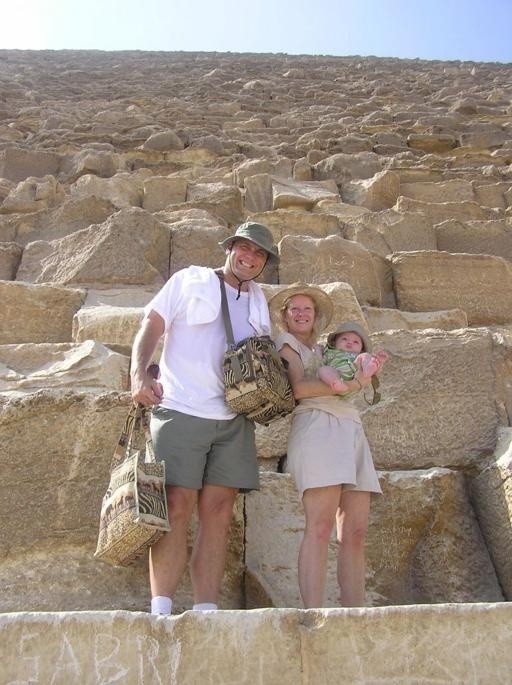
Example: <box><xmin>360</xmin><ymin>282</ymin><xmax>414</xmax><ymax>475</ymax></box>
<box><xmin>363</xmin><ymin>373</ymin><xmax>381</xmax><ymax>406</ymax></box>
<box><xmin>146</xmin><ymin>362</ymin><xmax>164</xmax><ymax>399</ymax></box>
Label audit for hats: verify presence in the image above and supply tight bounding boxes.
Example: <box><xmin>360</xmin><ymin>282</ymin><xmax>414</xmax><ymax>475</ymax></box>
<box><xmin>267</xmin><ymin>281</ymin><xmax>334</xmax><ymax>335</ymax></box>
<box><xmin>326</xmin><ymin>321</ymin><xmax>372</xmax><ymax>354</ymax></box>
<box><xmin>216</xmin><ymin>222</ymin><xmax>281</xmax><ymax>265</ymax></box>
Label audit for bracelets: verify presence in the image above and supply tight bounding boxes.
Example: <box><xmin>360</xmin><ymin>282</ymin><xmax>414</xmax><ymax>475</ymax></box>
<box><xmin>355</xmin><ymin>378</ymin><xmax>362</xmax><ymax>390</ymax></box>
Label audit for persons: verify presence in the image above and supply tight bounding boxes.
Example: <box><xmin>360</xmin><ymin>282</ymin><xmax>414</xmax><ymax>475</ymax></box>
<box><xmin>130</xmin><ymin>222</ymin><xmax>283</xmax><ymax>614</ymax></box>
<box><xmin>315</xmin><ymin>322</ymin><xmax>384</xmax><ymax>397</ymax></box>
<box><xmin>265</xmin><ymin>279</ymin><xmax>382</xmax><ymax>610</ymax></box>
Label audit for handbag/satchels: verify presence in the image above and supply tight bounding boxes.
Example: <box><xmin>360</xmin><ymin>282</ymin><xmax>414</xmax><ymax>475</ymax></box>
<box><xmin>94</xmin><ymin>402</ymin><xmax>173</xmax><ymax>569</ymax></box>
<box><xmin>224</xmin><ymin>335</ymin><xmax>295</xmax><ymax>426</ymax></box>
